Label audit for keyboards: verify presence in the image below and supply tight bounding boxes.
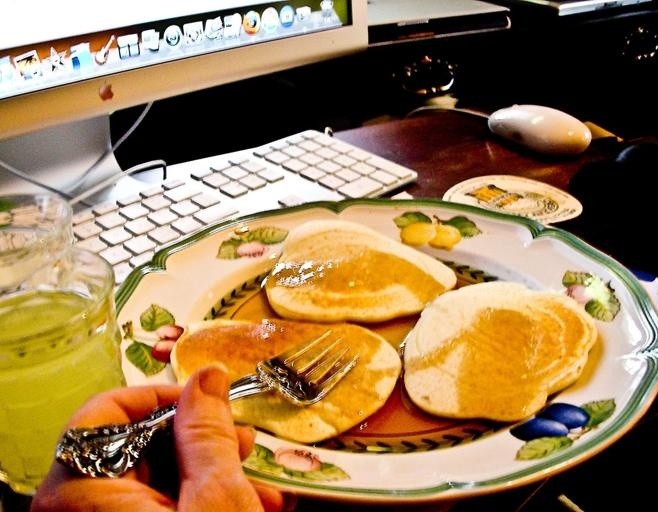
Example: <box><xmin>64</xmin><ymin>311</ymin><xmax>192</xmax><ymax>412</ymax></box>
<box><xmin>71</xmin><ymin>129</ymin><xmax>417</xmax><ymax>295</ymax></box>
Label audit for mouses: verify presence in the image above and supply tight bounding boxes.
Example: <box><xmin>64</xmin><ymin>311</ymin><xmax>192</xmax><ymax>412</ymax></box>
<box><xmin>487</xmin><ymin>104</ymin><xmax>592</xmax><ymax>158</ymax></box>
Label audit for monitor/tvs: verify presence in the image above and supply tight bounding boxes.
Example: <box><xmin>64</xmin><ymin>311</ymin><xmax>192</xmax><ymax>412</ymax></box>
<box><xmin>0</xmin><ymin>0</ymin><xmax>369</xmax><ymax>215</ymax></box>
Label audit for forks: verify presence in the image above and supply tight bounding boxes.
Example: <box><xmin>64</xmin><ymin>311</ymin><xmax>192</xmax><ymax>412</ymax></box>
<box><xmin>56</xmin><ymin>328</ymin><xmax>358</xmax><ymax>478</ymax></box>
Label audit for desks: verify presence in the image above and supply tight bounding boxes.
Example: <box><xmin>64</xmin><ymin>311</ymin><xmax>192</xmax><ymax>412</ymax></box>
<box><xmin>1</xmin><ymin>107</ymin><xmax>658</xmax><ymax>508</ymax></box>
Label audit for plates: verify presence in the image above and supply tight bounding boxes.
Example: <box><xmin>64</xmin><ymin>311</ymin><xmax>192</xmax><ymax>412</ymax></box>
<box><xmin>110</xmin><ymin>196</ymin><xmax>657</xmax><ymax>512</ymax></box>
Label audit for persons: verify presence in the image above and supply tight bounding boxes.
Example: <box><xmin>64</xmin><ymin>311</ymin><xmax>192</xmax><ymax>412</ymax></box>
<box><xmin>30</xmin><ymin>361</ymin><xmax>286</xmax><ymax>512</ymax></box>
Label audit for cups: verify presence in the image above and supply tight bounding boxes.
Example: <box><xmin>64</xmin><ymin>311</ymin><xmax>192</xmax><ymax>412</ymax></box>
<box><xmin>0</xmin><ymin>193</ymin><xmax>127</xmax><ymax>503</ymax></box>
